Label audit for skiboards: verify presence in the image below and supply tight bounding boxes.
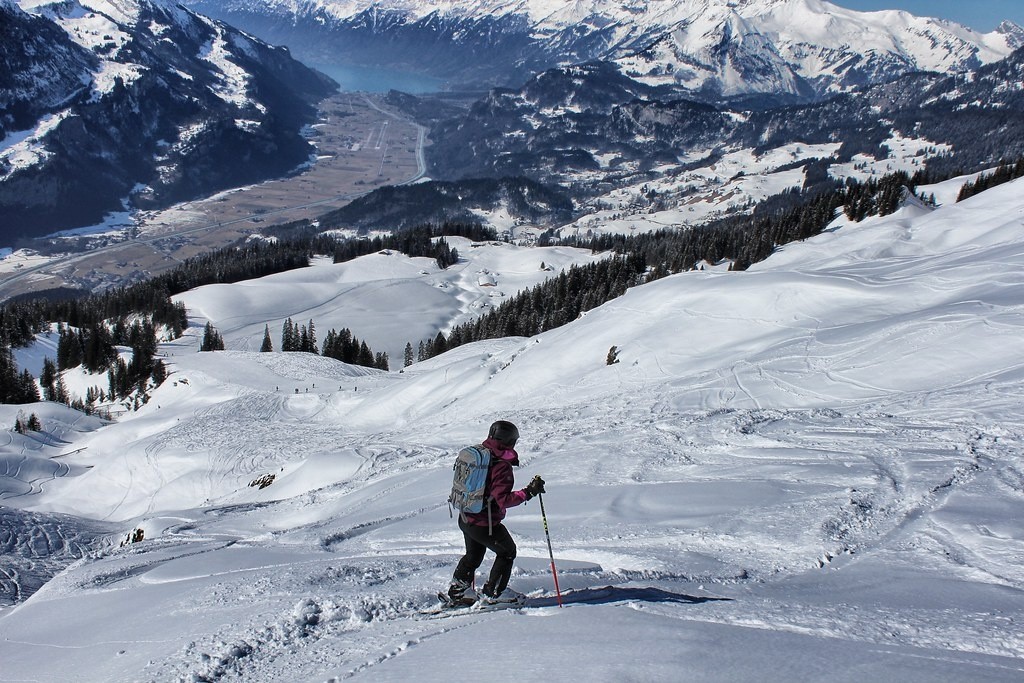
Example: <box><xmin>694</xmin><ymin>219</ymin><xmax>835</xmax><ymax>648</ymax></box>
<box><xmin>403</xmin><ymin>585</ymin><xmax>614</xmax><ymax>621</ymax></box>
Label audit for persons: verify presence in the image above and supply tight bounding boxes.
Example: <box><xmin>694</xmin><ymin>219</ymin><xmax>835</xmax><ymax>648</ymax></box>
<box><xmin>457</xmin><ymin>420</ymin><xmax>546</xmax><ymax>608</ymax></box>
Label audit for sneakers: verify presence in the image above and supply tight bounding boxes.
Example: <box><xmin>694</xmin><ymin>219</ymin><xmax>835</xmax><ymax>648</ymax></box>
<box><xmin>477</xmin><ymin>588</ymin><xmax>528</xmax><ymax>611</ymax></box>
<box><xmin>438</xmin><ymin>581</ymin><xmax>482</xmax><ymax>606</ymax></box>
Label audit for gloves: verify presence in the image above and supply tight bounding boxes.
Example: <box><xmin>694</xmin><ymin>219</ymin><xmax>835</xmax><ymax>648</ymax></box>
<box><xmin>523</xmin><ymin>476</ymin><xmax>545</xmax><ymax>501</ymax></box>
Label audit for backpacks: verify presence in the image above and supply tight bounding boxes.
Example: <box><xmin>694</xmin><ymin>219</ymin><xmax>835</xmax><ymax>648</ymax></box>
<box><xmin>447</xmin><ymin>446</ymin><xmax>492</xmax><ymax>514</ymax></box>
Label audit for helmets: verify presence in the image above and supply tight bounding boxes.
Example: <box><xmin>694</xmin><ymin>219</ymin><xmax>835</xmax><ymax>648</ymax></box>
<box><xmin>490</xmin><ymin>421</ymin><xmax>520</xmax><ymax>448</ymax></box>
